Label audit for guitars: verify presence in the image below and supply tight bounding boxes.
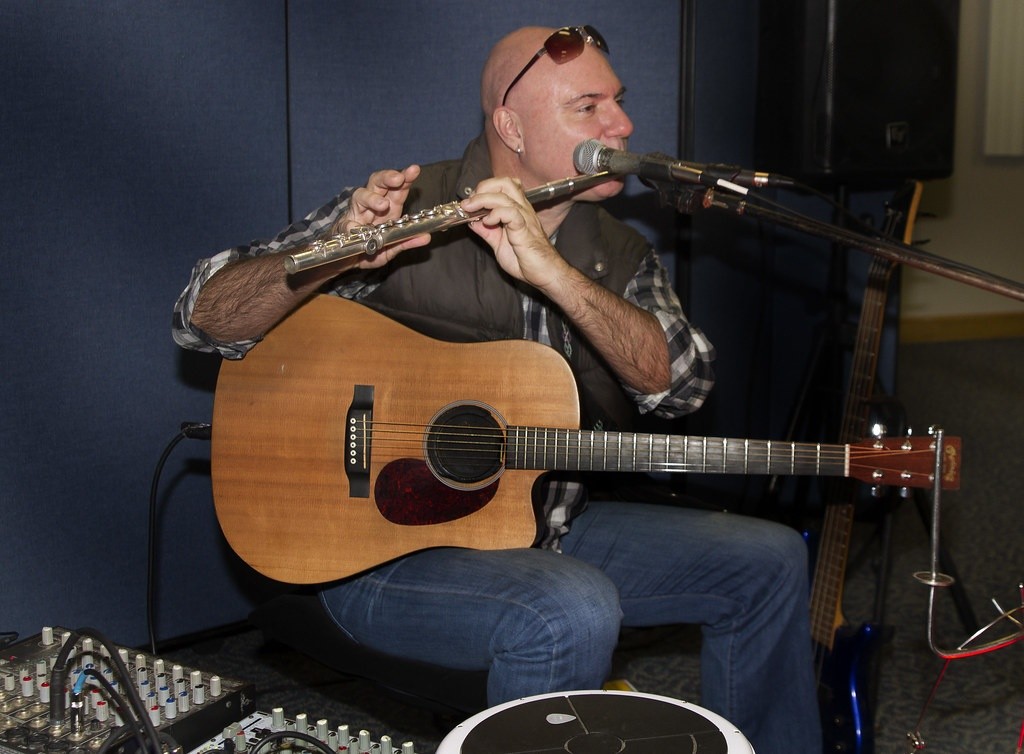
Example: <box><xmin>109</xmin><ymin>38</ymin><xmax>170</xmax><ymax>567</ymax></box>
<box><xmin>806</xmin><ymin>173</ymin><xmax>935</xmax><ymax>754</ymax></box>
<box><xmin>211</xmin><ymin>293</ymin><xmax>962</xmax><ymax>586</ymax></box>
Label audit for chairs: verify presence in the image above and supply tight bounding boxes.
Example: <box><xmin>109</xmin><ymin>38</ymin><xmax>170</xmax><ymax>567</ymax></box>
<box><xmin>243</xmin><ymin>176</ymin><xmax>976</xmax><ymax>754</ymax></box>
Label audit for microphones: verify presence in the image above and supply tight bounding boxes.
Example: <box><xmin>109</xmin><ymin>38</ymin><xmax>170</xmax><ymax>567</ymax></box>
<box><xmin>641</xmin><ymin>149</ymin><xmax>795</xmax><ymax>189</ymax></box>
<box><xmin>572</xmin><ymin>138</ymin><xmax>723</xmax><ymax>188</ymax></box>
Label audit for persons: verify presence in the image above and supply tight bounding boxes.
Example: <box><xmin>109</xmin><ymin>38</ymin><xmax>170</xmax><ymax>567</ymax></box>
<box><xmin>171</xmin><ymin>28</ymin><xmax>823</xmax><ymax>754</ymax></box>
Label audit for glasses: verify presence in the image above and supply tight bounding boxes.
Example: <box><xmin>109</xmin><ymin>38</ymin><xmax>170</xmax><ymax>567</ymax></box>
<box><xmin>502</xmin><ymin>24</ymin><xmax>610</xmax><ymax>109</ymax></box>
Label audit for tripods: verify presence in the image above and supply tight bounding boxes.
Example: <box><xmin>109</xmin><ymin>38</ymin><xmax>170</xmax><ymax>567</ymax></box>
<box><xmin>797</xmin><ymin>175</ymin><xmax>985</xmax><ymax>647</ymax></box>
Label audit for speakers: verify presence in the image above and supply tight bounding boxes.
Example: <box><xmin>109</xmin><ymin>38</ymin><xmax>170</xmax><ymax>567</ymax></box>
<box><xmin>753</xmin><ymin>0</ymin><xmax>957</xmax><ymax>193</ymax></box>
